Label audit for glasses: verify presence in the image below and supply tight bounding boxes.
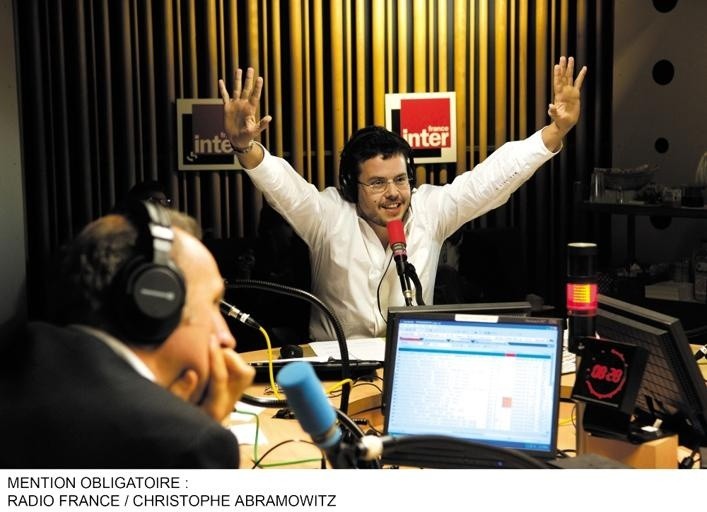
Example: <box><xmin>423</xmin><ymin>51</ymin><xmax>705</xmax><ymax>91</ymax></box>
<box><xmin>359</xmin><ymin>176</ymin><xmax>412</xmax><ymax>191</ymax></box>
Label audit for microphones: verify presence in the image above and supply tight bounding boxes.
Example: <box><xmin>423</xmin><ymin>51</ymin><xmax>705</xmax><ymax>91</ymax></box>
<box><xmin>222</xmin><ymin>300</ymin><xmax>261</xmax><ymax>333</ymax></box>
<box><xmin>564</xmin><ymin>241</ymin><xmax>599</xmax><ymax>356</ymax></box>
<box><xmin>386</xmin><ymin>218</ymin><xmax>413</xmax><ymax>300</ymax></box>
<box><xmin>276</xmin><ymin>361</ymin><xmax>361</xmax><ymax>469</ymax></box>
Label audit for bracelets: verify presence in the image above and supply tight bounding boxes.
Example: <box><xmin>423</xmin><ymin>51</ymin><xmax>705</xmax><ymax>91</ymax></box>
<box><xmin>228</xmin><ymin>139</ymin><xmax>252</xmax><ymax>153</ymax></box>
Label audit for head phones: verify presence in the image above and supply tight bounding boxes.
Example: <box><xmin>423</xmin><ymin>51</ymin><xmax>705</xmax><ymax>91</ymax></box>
<box><xmin>338</xmin><ymin>130</ymin><xmax>417</xmax><ymax>203</ymax></box>
<box><xmin>111</xmin><ymin>189</ymin><xmax>187</xmax><ymax>343</ymax></box>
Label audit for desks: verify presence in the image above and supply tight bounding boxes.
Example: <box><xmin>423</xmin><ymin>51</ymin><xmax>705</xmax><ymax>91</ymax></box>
<box><xmin>222</xmin><ymin>337</ymin><xmax>707</xmax><ymax>468</ymax></box>
<box><xmin>583</xmin><ymin>198</ymin><xmax>706</xmax><ymax>270</ymax></box>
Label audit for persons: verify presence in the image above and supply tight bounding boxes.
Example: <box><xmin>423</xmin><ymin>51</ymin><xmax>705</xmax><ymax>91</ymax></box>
<box><xmin>217</xmin><ymin>54</ymin><xmax>587</xmax><ymax>340</ymax></box>
<box><xmin>0</xmin><ymin>200</ymin><xmax>257</xmax><ymax>467</ymax></box>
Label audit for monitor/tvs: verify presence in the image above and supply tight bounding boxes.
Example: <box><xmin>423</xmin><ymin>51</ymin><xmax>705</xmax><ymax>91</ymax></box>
<box><xmin>383</xmin><ymin>312</ymin><xmax>563</xmax><ymax>460</ymax></box>
<box><xmin>379</xmin><ymin>300</ymin><xmax>555</xmax><ymax>416</ymax></box>
<box><xmin>596</xmin><ymin>294</ymin><xmax>706</xmax><ymax>448</ymax></box>
